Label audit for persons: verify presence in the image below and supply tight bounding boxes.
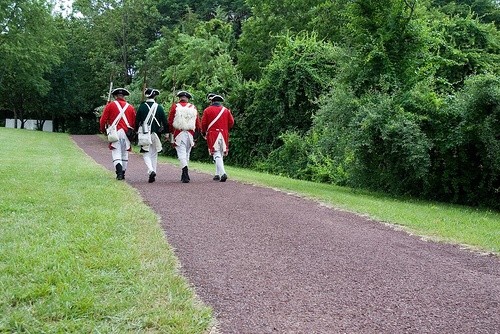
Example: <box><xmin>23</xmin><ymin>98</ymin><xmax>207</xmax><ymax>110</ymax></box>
<box><xmin>100</xmin><ymin>88</ymin><xmax>136</xmax><ymax>180</ymax></box>
<box><xmin>135</xmin><ymin>88</ymin><xmax>169</xmax><ymax>183</ymax></box>
<box><xmin>201</xmin><ymin>93</ymin><xmax>234</xmax><ymax>182</ymax></box>
<box><xmin>168</xmin><ymin>91</ymin><xmax>201</xmax><ymax>183</ymax></box>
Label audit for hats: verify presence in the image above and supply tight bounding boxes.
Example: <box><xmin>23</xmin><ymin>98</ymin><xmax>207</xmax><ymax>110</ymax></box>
<box><xmin>208</xmin><ymin>94</ymin><xmax>225</xmax><ymax>102</ymax></box>
<box><xmin>112</xmin><ymin>88</ymin><xmax>130</xmax><ymax>95</ymax></box>
<box><xmin>176</xmin><ymin>92</ymin><xmax>194</xmax><ymax>101</ymax></box>
<box><xmin>144</xmin><ymin>88</ymin><xmax>159</xmax><ymax>98</ymax></box>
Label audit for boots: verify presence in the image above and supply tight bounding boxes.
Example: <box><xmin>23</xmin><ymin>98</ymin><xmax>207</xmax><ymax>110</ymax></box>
<box><xmin>122</xmin><ymin>170</ymin><xmax>125</xmax><ymax>179</ymax></box>
<box><xmin>183</xmin><ymin>166</ymin><xmax>189</xmax><ymax>183</ymax></box>
<box><xmin>116</xmin><ymin>164</ymin><xmax>124</xmax><ymax>180</ymax></box>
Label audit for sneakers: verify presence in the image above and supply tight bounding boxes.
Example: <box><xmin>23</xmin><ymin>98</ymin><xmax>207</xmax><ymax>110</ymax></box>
<box><xmin>213</xmin><ymin>175</ymin><xmax>220</xmax><ymax>180</ymax></box>
<box><xmin>220</xmin><ymin>174</ymin><xmax>228</xmax><ymax>182</ymax></box>
<box><xmin>149</xmin><ymin>171</ymin><xmax>156</xmax><ymax>183</ymax></box>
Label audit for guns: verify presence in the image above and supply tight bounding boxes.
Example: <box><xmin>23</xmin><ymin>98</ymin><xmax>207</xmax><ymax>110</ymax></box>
<box><xmin>169</xmin><ymin>75</ymin><xmax>177</xmax><ymax>146</ymax></box>
<box><xmin>141</xmin><ymin>69</ymin><xmax>148</xmax><ymax>105</ymax></box>
<box><xmin>104</xmin><ymin>81</ymin><xmax>114</xmax><ymax>137</ymax></box>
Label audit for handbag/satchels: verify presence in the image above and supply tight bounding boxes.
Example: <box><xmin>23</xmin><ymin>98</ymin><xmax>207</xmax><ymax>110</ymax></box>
<box><xmin>106</xmin><ymin>125</ymin><xmax>119</xmax><ymax>142</ymax></box>
<box><xmin>138</xmin><ymin>126</ymin><xmax>152</xmax><ymax>145</ymax></box>
